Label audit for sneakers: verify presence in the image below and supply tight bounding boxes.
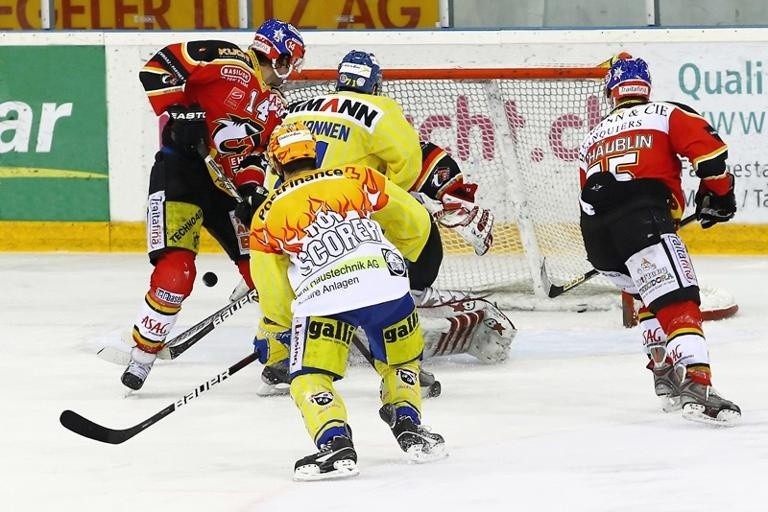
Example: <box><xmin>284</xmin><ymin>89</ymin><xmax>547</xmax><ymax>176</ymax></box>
<box><xmin>647</xmin><ymin>342</ymin><xmax>678</xmax><ymax>396</ymax></box>
<box><xmin>263</xmin><ymin>360</ymin><xmax>293</xmax><ymax>386</ymax></box>
<box><xmin>677</xmin><ymin>365</ymin><xmax>742</xmax><ymax>415</ymax></box>
<box><xmin>293</xmin><ymin>423</ymin><xmax>357</xmax><ymax>471</ymax></box>
<box><xmin>379</xmin><ymin>404</ymin><xmax>444</xmax><ymax>449</ymax></box>
<box><xmin>120</xmin><ymin>347</ymin><xmax>157</xmax><ymax>389</ymax></box>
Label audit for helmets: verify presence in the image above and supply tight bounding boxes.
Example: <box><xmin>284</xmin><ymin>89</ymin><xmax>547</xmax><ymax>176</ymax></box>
<box><xmin>249</xmin><ymin>19</ymin><xmax>305</xmax><ymax>78</ymax></box>
<box><xmin>336</xmin><ymin>48</ymin><xmax>382</xmax><ymax>95</ymax></box>
<box><xmin>268</xmin><ymin>120</ymin><xmax>317</xmax><ymax>174</ymax></box>
<box><xmin>604</xmin><ymin>59</ymin><xmax>652</xmax><ymax>100</ymax></box>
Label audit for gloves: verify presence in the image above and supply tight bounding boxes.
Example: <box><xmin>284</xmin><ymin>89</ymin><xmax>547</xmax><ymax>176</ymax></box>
<box><xmin>234</xmin><ymin>182</ymin><xmax>267</xmax><ymax>224</ymax></box>
<box><xmin>693</xmin><ymin>172</ymin><xmax>735</xmax><ymax>229</ymax></box>
<box><xmin>161</xmin><ymin>103</ymin><xmax>208</xmax><ymax>149</ymax></box>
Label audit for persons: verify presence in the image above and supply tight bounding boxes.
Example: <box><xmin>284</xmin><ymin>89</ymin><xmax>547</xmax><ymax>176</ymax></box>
<box><xmin>348</xmin><ymin>140</ymin><xmax>516</xmax><ymax>368</ymax></box>
<box><xmin>262</xmin><ymin>49</ymin><xmax>435</xmax><ymax>389</ymax></box>
<box><xmin>249</xmin><ymin>122</ymin><xmax>446</xmax><ymax>482</ymax></box>
<box><xmin>579</xmin><ymin>57</ymin><xmax>742</xmax><ymax>428</ymax></box>
<box><xmin>121</xmin><ymin>19</ymin><xmax>306</xmax><ymax>391</ymax></box>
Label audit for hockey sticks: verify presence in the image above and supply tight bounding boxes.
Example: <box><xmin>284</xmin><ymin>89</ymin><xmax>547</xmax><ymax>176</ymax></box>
<box><xmin>541</xmin><ymin>212</ymin><xmax>697</xmax><ymax>298</ymax></box>
<box><xmin>95</xmin><ymin>289</ymin><xmax>259</xmax><ymax>366</ymax></box>
<box><xmin>60</xmin><ymin>351</ymin><xmax>260</xmax><ymax>444</ymax></box>
